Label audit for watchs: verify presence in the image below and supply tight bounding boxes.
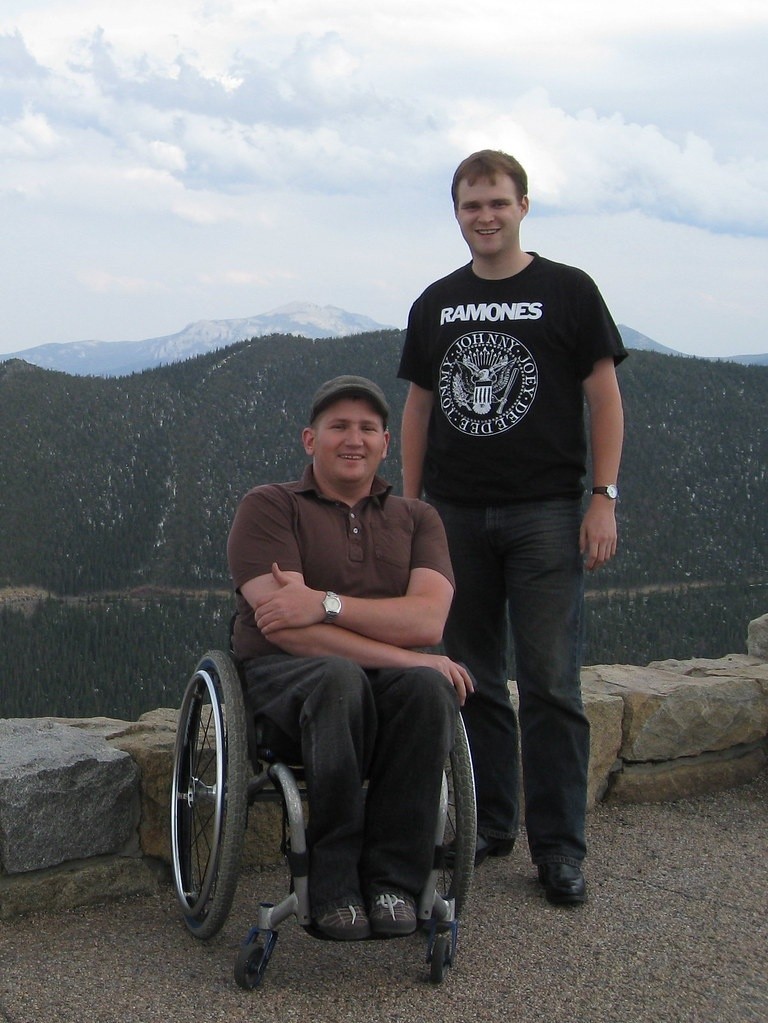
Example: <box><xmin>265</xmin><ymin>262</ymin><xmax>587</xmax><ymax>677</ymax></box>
<box><xmin>322</xmin><ymin>590</ymin><xmax>342</xmax><ymax>624</ymax></box>
<box><xmin>591</xmin><ymin>484</ymin><xmax>620</xmax><ymax>500</ymax></box>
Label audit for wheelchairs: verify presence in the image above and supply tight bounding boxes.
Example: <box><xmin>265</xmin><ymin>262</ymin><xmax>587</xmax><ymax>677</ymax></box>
<box><xmin>165</xmin><ymin>608</ymin><xmax>475</xmax><ymax>990</ymax></box>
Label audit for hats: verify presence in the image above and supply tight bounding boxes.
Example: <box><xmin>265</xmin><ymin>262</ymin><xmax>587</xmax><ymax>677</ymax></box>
<box><xmin>309</xmin><ymin>374</ymin><xmax>390</xmax><ymax>424</ymax></box>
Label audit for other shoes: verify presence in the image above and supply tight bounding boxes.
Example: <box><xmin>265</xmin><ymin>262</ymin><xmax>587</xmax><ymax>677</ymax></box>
<box><xmin>312</xmin><ymin>904</ymin><xmax>373</xmax><ymax>940</ymax></box>
<box><xmin>368</xmin><ymin>888</ymin><xmax>418</xmax><ymax>934</ymax></box>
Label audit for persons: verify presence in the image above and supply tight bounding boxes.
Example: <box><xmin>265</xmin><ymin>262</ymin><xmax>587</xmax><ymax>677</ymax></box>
<box><xmin>397</xmin><ymin>149</ymin><xmax>629</xmax><ymax>905</ymax></box>
<box><xmin>227</xmin><ymin>375</ymin><xmax>475</xmax><ymax>942</ymax></box>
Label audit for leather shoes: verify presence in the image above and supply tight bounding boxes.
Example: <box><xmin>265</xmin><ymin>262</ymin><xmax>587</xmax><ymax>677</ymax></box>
<box><xmin>434</xmin><ymin>831</ymin><xmax>516</xmax><ymax>866</ymax></box>
<box><xmin>537</xmin><ymin>862</ymin><xmax>587</xmax><ymax>904</ymax></box>
